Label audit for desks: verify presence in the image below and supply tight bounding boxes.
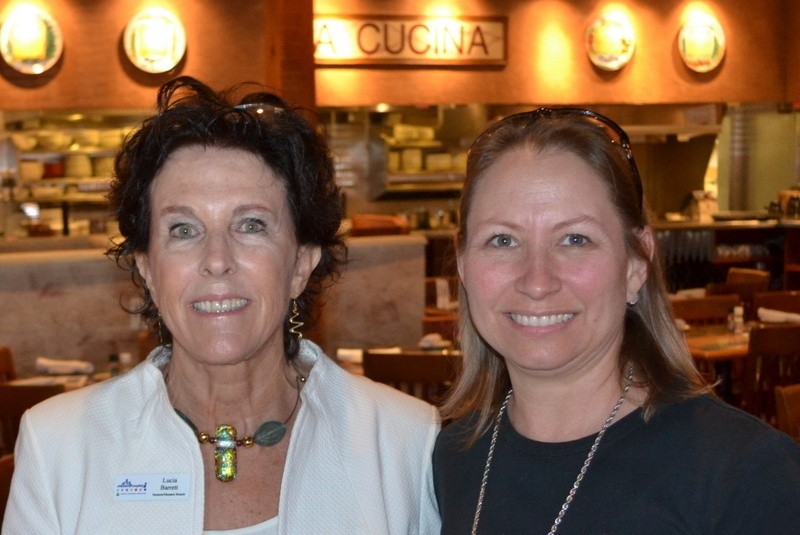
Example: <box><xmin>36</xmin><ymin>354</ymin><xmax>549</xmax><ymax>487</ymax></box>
<box><xmin>0</xmin><ymin>365</ymin><xmax>138</xmax><ymax>393</ymax></box>
<box><xmin>668</xmin><ymin>319</ymin><xmax>759</xmax><ymax>412</ymax></box>
<box><xmin>321</xmin><ymin>342</ymin><xmax>457</xmax><ymax>379</ymax></box>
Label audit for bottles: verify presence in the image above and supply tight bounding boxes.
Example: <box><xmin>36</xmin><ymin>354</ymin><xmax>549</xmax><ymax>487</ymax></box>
<box><xmin>735</xmin><ymin>306</ymin><xmax>744</xmax><ymax>334</ymax></box>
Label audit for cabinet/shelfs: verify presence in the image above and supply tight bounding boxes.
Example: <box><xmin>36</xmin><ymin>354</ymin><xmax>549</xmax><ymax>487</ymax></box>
<box><xmin>0</xmin><ymin>109</ymin><xmax>156</xmax><ymax>245</ymax></box>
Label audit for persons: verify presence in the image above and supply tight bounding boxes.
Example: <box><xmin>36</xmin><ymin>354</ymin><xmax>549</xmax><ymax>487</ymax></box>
<box><xmin>349</xmin><ymin>112</ymin><xmax>389</xmax><ymax>201</ymax></box>
<box><xmin>433</xmin><ymin>108</ymin><xmax>800</xmax><ymax>535</ymax></box>
<box><xmin>0</xmin><ymin>75</ymin><xmax>441</xmax><ymax>535</ymax></box>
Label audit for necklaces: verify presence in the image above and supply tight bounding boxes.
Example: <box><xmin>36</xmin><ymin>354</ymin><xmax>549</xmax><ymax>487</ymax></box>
<box><xmin>472</xmin><ymin>366</ymin><xmax>634</xmax><ymax>535</ymax></box>
<box><xmin>164</xmin><ymin>360</ymin><xmax>307</xmax><ymax>482</ymax></box>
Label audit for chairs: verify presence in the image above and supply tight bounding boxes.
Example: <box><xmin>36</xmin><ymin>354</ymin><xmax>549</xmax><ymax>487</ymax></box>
<box><xmin>0</xmin><ymin>267</ymin><xmax>800</xmax><ymax>528</ymax></box>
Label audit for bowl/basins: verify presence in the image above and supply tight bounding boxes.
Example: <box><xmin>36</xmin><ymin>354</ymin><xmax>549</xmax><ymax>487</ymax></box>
<box><xmin>11</xmin><ymin>127</ymin><xmax>136</xmax><ymax>180</ymax></box>
<box><xmin>386</xmin><ymin>149</ymin><xmax>470</xmax><ymax>173</ymax></box>
<box><xmin>395</xmin><ymin>206</ymin><xmax>460</xmax><ymax>229</ymax></box>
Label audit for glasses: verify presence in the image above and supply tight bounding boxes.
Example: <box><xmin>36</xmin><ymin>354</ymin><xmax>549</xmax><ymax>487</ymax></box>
<box><xmin>158</xmin><ymin>102</ymin><xmax>286</xmax><ymax>121</ymax></box>
<box><xmin>468</xmin><ymin>107</ymin><xmax>632</xmax><ymax>159</ymax></box>
